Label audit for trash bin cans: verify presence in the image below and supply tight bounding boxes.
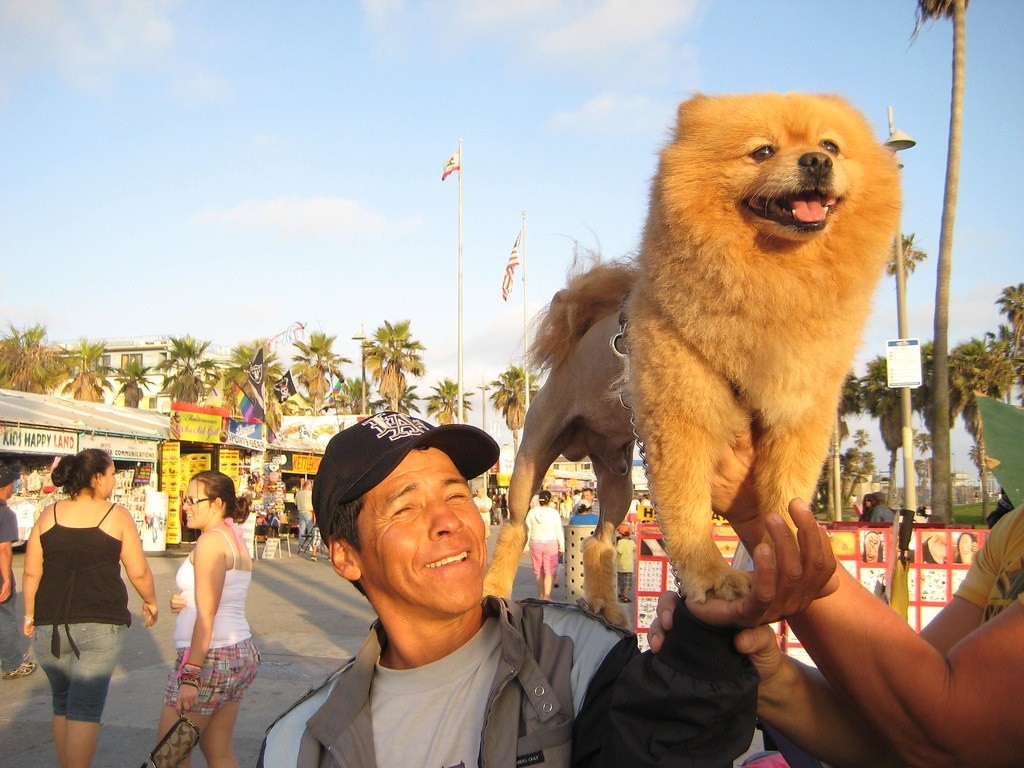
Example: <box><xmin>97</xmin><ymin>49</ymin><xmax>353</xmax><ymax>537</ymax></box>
<box><xmin>563</xmin><ymin>514</ymin><xmax>600</xmax><ymax>606</ymax></box>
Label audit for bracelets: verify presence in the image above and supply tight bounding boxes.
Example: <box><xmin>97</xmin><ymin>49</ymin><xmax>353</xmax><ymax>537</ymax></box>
<box><xmin>177</xmin><ymin>663</ymin><xmax>202</xmax><ymax>690</ymax></box>
<box><xmin>24</xmin><ymin>616</ymin><xmax>34</xmax><ymax>624</ymax></box>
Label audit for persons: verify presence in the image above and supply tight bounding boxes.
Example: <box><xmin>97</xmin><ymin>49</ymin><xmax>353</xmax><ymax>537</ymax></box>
<box><xmin>887</xmin><ymin>503</ymin><xmax>908</xmax><ymax>516</ymax></box>
<box><xmin>310</xmin><ymin>524</ymin><xmax>321</xmax><ymax>562</ymax></box>
<box><xmin>156</xmin><ymin>470</ymin><xmax>262</xmax><ymax>768</ymax></box>
<box><xmin>256</xmin><ymin>410</ymin><xmax>840</xmax><ymax>768</ymax></box>
<box><xmin>470</xmin><ymin>485</ymin><xmax>659</xmax><ymax>603</ymax></box>
<box><xmin>295</xmin><ymin>479</ymin><xmax>313</xmax><ymax>552</ymax></box>
<box><xmin>0</xmin><ymin>469</ymin><xmax>36</xmax><ymax>680</ymax></box>
<box><xmin>851</xmin><ymin>492</ymin><xmax>895</xmax><ymax>522</ymax></box>
<box><xmin>986</xmin><ymin>487</ymin><xmax>1016</xmax><ymax>529</ymax></box>
<box><xmin>22</xmin><ymin>449</ymin><xmax>158</xmax><ymax>768</ymax></box>
<box><xmin>646</xmin><ymin>495</ymin><xmax>1024</xmax><ymax>768</ymax></box>
<box><xmin>917</xmin><ymin>503</ymin><xmax>931</xmax><ymax>518</ymax></box>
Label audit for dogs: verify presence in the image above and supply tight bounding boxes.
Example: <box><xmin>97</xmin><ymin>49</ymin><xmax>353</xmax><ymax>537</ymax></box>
<box><xmin>486</xmin><ymin>91</ymin><xmax>902</xmax><ymax>606</ymax></box>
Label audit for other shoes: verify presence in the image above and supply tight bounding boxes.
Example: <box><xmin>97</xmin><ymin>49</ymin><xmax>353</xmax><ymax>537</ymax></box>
<box><xmin>298</xmin><ymin>546</ymin><xmax>306</xmax><ymax>551</ymax></box>
<box><xmin>308</xmin><ymin>545</ymin><xmax>313</xmax><ymax>551</ymax></box>
<box><xmin>554</xmin><ymin>584</ymin><xmax>559</xmax><ymax>589</ymax></box>
<box><xmin>310</xmin><ymin>556</ymin><xmax>317</xmax><ymax>561</ymax></box>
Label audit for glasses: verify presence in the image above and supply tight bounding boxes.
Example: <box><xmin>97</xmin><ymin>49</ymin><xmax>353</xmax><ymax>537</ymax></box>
<box><xmin>183</xmin><ymin>496</ymin><xmax>215</xmax><ymax>506</ymax></box>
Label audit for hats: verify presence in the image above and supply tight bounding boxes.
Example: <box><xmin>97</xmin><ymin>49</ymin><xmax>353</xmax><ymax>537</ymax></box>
<box><xmin>618</xmin><ymin>524</ymin><xmax>630</xmax><ymax>535</ymax></box>
<box><xmin>872</xmin><ymin>492</ymin><xmax>885</xmax><ymax>501</ymax></box>
<box><xmin>577</xmin><ymin>498</ymin><xmax>590</xmax><ymax>510</ymax></box>
<box><xmin>0</xmin><ymin>465</ymin><xmax>21</xmax><ymax>487</ymax></box>
<box><xmin>311</xmin><ymin>411</ymin><xmax>501</xmax><ymax>546</ymax></box>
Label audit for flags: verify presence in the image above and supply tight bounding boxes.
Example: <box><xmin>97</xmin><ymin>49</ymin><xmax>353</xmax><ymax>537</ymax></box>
<box><xmin>502</xmin><ymin>228</ymin><xmax>522</xmax><ymax>302</ymax></box>
<box><xmin>232</xmin><ymin>383</ymin><xmax>255</xmax><ymax>423</ymax></box>
<box><xmin>442</xmin><ymin>147</ymin><xmax>460</xmax><ymax>181</ymax></box>
<box><xmin>273</xmin><ymin>370</ymin><xmax>297</xmax><ymax>403</ymax></box>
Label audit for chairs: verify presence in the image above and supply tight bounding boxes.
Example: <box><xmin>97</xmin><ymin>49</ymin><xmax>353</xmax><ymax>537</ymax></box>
<box><xmin>254</xmin><ymin>521</ymin><xmax>318</xmax><ymax>561</ymax></box>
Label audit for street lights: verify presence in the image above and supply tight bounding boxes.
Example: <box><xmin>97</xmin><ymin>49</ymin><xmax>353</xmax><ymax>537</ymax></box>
<box><xmin>351</xmin><ymin>322</ymin><xmax>367</xmax><ymax>414</ymax></box>
<box><xmin>880</xmin><ymin>103</ymin><xmax>926</xmax><ymax>524</ymax></box>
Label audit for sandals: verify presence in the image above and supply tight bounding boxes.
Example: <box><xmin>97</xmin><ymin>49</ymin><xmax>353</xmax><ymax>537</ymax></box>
<box><xmin>624</xmin><ymin>597</ymin><xmax>632</xmax><ymax>603</ymax></box>
<box><xmin>618</xmin><ymin>594</ymin><xmax>624</xmax><ymax>601</ymax></box>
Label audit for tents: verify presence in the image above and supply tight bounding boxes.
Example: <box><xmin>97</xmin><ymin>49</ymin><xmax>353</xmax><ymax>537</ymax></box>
<box><xmin>0</xmin><ymin>387</ymin><xmax>171</xmax><ymax>553</ymax></box>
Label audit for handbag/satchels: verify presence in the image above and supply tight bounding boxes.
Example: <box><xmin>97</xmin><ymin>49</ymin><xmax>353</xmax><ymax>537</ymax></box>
<box><xmin>140</xmin><ymin>682</ymin><xmax>199</xmax><ymax>768</ymax></box>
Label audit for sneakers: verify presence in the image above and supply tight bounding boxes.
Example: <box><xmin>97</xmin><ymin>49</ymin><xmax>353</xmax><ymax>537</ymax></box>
<box><xmin>3</xmin><ymin>658</ymin><xmax>35</xmax><ymax>680</ymax></box>
